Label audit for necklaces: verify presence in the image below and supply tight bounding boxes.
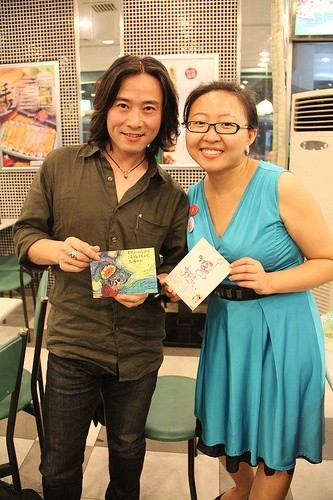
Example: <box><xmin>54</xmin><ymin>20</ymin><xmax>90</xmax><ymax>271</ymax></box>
<box><xmin>104</xmin><ymin>146</ymin><xmax>146</xmax><ymax>179</ymax></box>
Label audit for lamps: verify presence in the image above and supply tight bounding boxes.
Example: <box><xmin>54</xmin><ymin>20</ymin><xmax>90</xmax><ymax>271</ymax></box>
<box><xmin>256</xmin><ymin>64</ymin><xmax>274</xmax><ymax>113</ymax></box>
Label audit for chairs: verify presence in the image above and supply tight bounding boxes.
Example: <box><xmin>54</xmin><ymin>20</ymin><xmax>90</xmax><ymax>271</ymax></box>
<box><xmin>0</xmin><ymin>327</ymin><xmax>29</xmax><ymax>493</ymax></box>
<box><xmin>0</xmin><ymin>270</ymin><xmax>50</xmax><ymax>454</ymax></box>
<box><xmin>0</xmin><ymin>265</ymin><xmax>37</xmax><ymax>343</ymax></box>
<box><xmin>144</xmin><ymin>374</ymin><xmax>198</xmax><ymax>500</ymax></box>
<box><xmin>0</xmin><ymin>252</ymin><xmax>41</xmax><ymax>299</ymax></box>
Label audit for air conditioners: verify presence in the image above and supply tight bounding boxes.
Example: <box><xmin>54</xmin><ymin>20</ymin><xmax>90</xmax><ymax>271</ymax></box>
<box><xmin>288</xmin><ymin>86</ymin><xmax>333</xmax><ymax>316</ymax></box>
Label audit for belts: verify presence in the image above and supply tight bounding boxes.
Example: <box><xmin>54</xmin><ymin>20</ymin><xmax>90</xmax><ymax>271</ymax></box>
<box><xmin>212</xmin><ymin>284</ymin><xmax>277</xmax><ymax>301</ymax></box>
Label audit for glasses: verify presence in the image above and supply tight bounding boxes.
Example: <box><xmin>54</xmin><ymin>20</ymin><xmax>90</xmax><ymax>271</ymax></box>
<box><xmin>185</xmin><ymin>121</ymin><xmax>248</xmax><ymax>134</ymax></box>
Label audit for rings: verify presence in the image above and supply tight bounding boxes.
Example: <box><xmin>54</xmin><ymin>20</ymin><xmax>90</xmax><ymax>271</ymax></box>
<box><xmin>69</xmin><ymin>250</ymin><xmax>77</xmax><ymax>260</ymax></box>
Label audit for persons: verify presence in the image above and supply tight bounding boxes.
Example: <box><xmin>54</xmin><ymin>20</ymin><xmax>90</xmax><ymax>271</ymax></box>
<box><xmin>164</xmin><ymin>81</ymin><xmax>333</xmax><ymax>500</ymax></box>
<box><xmin>12</xmin><ymin>55</ymin><xmax>189</xmax><ymax>500</ymax></box>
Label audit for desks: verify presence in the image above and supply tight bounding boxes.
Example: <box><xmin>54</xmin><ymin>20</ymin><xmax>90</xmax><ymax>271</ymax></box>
<box><xmin>0</xmin><ymin>218</ymin><xmax>19</xmax><ymax>232</ymax></box>
<box><xmin>316</xmin><ymin>311</ymin><xmax>333</xmax><ymax>396</ymax></box>
<box><xmin>0</xmin><ymin>295</ymin><xmax>22</xmax><ymax>323</ymax></box>
